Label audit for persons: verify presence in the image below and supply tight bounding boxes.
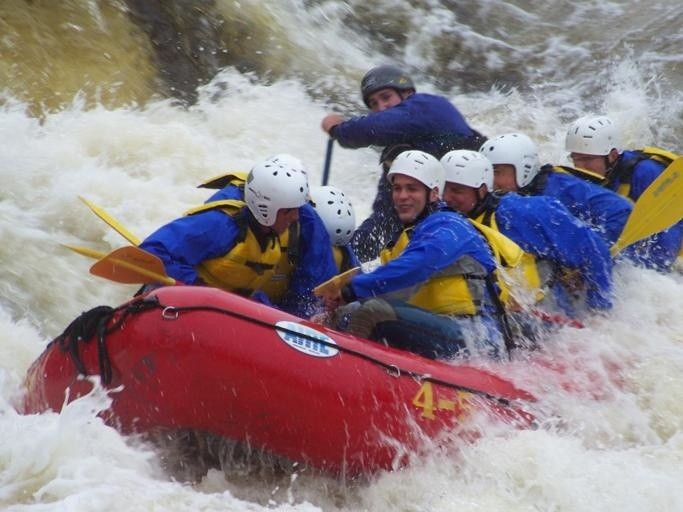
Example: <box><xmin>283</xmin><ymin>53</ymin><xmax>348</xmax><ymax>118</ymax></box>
<box><xmin>566</xmin><ymin>116</ymin><xmax>682</xmax><ymax>270</ymax></box>
<box><xmin>321</xmin><ymin>66</ymin><xmax>495</xmax><ymax>266</ymax></box>
<box><xmin>133</xmin><ymin>154</ymin><xmax>339</xmax><ymax>322</ymax></box>
<box><xmin>439</xmin><ymin>149</ymin><xmax>614</xmax><ymax>319</ymax></box>
<box><xmin>315</xmin><ymin>150</ymin><xmax>516</xmax><ymax>361</ymax></box>
<box><xmin>311</xmin><ymin>186</ymin><xmax>368</xmax><ymax>304</ymax></box>
<box><xmin>479</xmin><ymin>133</ymin><xmax>654</xmax><ymax>270</ymax></box>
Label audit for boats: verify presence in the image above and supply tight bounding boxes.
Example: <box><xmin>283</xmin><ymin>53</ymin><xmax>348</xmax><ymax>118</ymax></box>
<box><xmin>13</xmin><ymin>285</ymin><xmax>643</xmax><ymax>489</ymax></box>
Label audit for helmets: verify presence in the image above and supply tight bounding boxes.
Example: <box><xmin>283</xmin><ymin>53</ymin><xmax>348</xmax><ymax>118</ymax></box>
<box><xmin>564</xmin><ymin>114</ymin><xmax>626</xmax><ymax>179</ymax></box>
<box><xmin>359</xmin><ymin>64</ymin><xmax>417</xmax><ymax>109</ymax></box>
<box><xmin>245</xmin><ymin>153</ymin><xmax>311</xmax><ymax>228</ymax></box>
<box><xmin>312</xmin><ymin>186</ymin><xmax>356</xmax><ymax>248</ymax></box>
<box><xmin>439</xmin><ymin>149</ymin><xmax>494</xmax><ymax>216</ymax></box>
<box><xmin>477</xmin><ymin>133</ymin><xmax>543</xmax><ymax>191</ymax></box>
<box><xmin>385</xmin><ymin>149</ymin><xmax>448</xmax><ymax>226</ymax></box>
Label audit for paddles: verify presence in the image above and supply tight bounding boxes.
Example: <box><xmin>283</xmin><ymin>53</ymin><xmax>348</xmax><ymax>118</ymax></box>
<box><xmin>312</xmin><ymin>267</ymin><xmax>361</xmax><ymax>312</ymax></box>
<box><xmin>90</xmin><ymin>246</ymin><xmax>190</xmax><ymax>287</ymax></box>
<box><xmin>77</xmin><ymin>193</ymin><xmax>141</xmax><ymax>248</ymax></box>
<box><xmin>58</xmin><ymin>241</ymin><xmax>114</xmax><ymax>260</ymax></box>
<box><xmin>545</xmin><ymin>156</ymin><xmax>683</xmax><ymax>283</ymax></box>
